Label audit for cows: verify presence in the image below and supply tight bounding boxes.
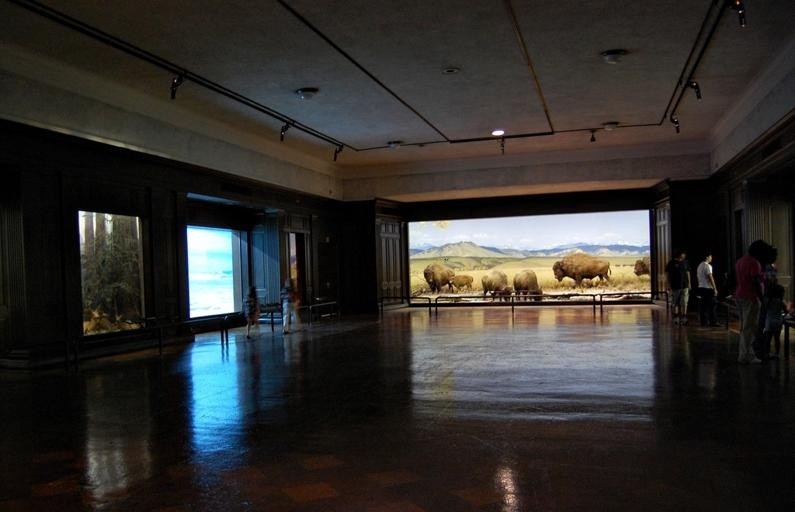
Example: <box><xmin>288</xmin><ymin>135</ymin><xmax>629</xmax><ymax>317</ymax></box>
<box><xmin>447</xmin><ymin>275</ymin><xmax>474</xmax><ymax>293</ymax></box>
<box><xmin>423</xmin><ymin>262</ymin><xmax>455</xmax><ymax>295</ymax></box>
<box><xmin>552</xmin><ymin>253</ymin><xmax>614</xmax><ymax>293</ymax></box>
<box><xmin>480</xmin><ymin>269</ymin><xmax>513</xmax><ymax>302</ymax></box>
<box><xmin>513</xmin><ymin>269</ymin><xmax>543</xmax><ymax>301</ymax></box>
<box><xmin>633</xmin><ymin>256</ymin><xmax>651</xmax><ymax>277</ymax></box>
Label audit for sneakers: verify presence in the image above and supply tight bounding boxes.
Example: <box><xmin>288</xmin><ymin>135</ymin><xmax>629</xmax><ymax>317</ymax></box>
<box><xmin>738</xmin><ymin>350</ymin><xmax>780</xmax><ymax>364</ymax></box>
<box><xmin>673</xmin><ymin>316</ymin><xmax>722</xmax><ymax>327</ymax></box>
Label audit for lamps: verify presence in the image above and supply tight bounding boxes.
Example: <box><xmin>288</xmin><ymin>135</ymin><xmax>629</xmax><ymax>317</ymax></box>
<box><xmin>670</xmin><ymin>115</ymin><xmax>680</xmax><ymax>134</ymax></box>
<box><xmin>600</xmin><ymin>49</ymin><xmax>632</xmax><ymax>65</ymax></box>
<box><xmin>333</xmin><ymin>144</ymin><xmax>343</xmax><ymax>162</ymax></box>
<box><xmin>387</xmin><ymin>140</ymin><xmax>402</xmax><ymax>149</ymax></box>
<box><xmin>688</xmin><ymin>80</ymin><xmax>702</xmax><ymax>102</ymax></box>
<box><xmin>280</xmin><ymin>121</ymin><xmax>291</xmax><ymax>142</ymax></box>
<box><xmin>732</xmin><ymin>0</ymin><xmax>746</xmax><ymax>29</ymax></box>
<box><xmin>601</xmin><ymin>122</ymin><xmax>619</xmax><ymax>131</ymax></box>
<box><xmin>296</xmin><ymin>88</ymin><xmax>318</xmax><ymax>101</ymax></box>
<box><xmin>170</xmin><ymin>72</ymin><xmax>184</xmax><ymax>99</ymax></box>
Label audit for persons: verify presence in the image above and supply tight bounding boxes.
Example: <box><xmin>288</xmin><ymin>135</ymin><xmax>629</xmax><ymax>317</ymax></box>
<box><xmin>281</xmin><ymin>278</ymin><xmax>295</xmax><ymax>334</ymax></box>
<box><xmin>753</xmin><ymin>247</ymin><xmax>778</xmax><ymax>359</ymax></box>
<box><xmin>287</xmin><ymin>287</ymin><xmax>304</xmax><ymax>331</ymax></box>
<box><xmin>243</xmin><ymin>286</ymin><xmax>260</xmax><ymax>338</ymax></box>
<box><xmin>761</xmin><ymin>284</ymin><xmax>788</xmax><ymax>364</ymax></box>
<box><xmin>696</xmin><ymin>253</ymin><xmax>726</xmax><ymax>326</ymax></box>
<box><xmin>735</xmin><ymin>239</ymin><xmax>772</xmax><ymax>366</ymax></box>
<box><xmin>664</xmin><ymin>253</ymin><xmax>692</xmax><ymax>325</ymax></box>
